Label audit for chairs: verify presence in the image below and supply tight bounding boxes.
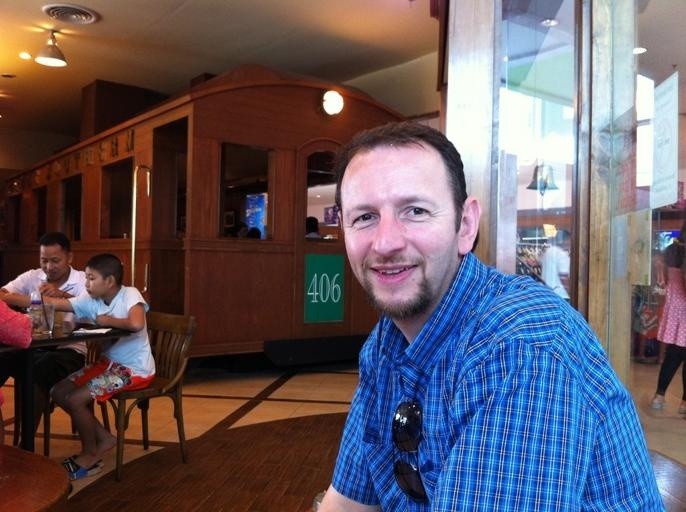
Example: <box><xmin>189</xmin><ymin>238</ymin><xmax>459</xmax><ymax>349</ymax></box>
<box><xmin>0</xmin><ymin>445</ymin><xmax>71</xmax><ymax>512</ymax></box>
<box><xmin>87</xmin><ymin>309</ymin><xmax>197</xmax><ymax>481</ymax></box>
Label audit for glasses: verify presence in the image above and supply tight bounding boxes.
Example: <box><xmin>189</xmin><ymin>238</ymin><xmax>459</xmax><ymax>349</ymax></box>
<box><xmin>392</xmin><ymin>399</ymin><xmax>429</xmax><ymax>505</ymax></box>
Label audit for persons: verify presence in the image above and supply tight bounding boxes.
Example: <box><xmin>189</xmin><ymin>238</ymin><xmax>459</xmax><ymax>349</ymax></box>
<box><xmin>0</xmin><ymin>300</ymin><xmax>32</xmax><ymax>385</ymax></box>
<box><xmin>631</xmin><ymin>225</ymin><xmax>686</xmax><ymax>414</ymax></box>
<box><xmin>224</xmin><ymin>222</ymin><xmax>261</xmax><ymax>240</ymax></box>
<box><xmin>1</xmin><ymin>232</ymin><xmax>88</xmax><ymax>450</ymax></box>
<box><xmin>4</xmin><ymin>253</ymin><xmax>156</xmax><ymax>481</ymax></box>
<box><xmin>315</xmin><ymin>122</ymin><xmax>669</xmax><ymax>512</ymax></box>
<box><xmin>305</xmin><ymin>217</ymin><xmax>319</xmax><ymax>238</ymax></box>
<box><xmin>542</xmin><ymin>229</ymin><xmax>572</xmax><ymax>302</ymax></box>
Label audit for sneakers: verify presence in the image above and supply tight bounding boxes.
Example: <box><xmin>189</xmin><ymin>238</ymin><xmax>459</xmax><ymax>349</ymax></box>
<box><xmin>649</xmin><ymin>397</ymin><xmax>686</xmax><ymax>413</ymax></box>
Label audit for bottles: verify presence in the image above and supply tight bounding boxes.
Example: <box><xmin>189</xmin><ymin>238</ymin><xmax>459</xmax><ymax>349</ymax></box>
<box><xmin>30</xmin><ymin>290</ymin><xmax>42</xmax><ymax>333</ymax></box>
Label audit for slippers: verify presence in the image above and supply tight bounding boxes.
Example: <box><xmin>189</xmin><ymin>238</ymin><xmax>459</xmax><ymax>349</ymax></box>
<box><xmin>63</xmin><ymin>454</ymin><xmax>104</xmax><ymax>466</ymax></box>
<box><xmin>62</xmin><ymin>462</ymin><xmax>101</xmax><ymax>480</ymax></box>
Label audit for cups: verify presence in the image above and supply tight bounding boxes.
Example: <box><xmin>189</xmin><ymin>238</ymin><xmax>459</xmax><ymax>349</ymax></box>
<box><xmin>40</xmin><ymin>301</ymin><xmax>55</xmax><ymax>335</ymax></box>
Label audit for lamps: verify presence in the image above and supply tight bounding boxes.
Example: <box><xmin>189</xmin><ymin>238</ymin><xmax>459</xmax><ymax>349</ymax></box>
<box><xmin>321</xmin><ymin>90</ymin><xmax>344</xmax><ymax>116</ymax></box>
<box><xmin>35</xmin><ymin>30</ymin><xmax>68</xmax><ymax>68</ymax></box>
